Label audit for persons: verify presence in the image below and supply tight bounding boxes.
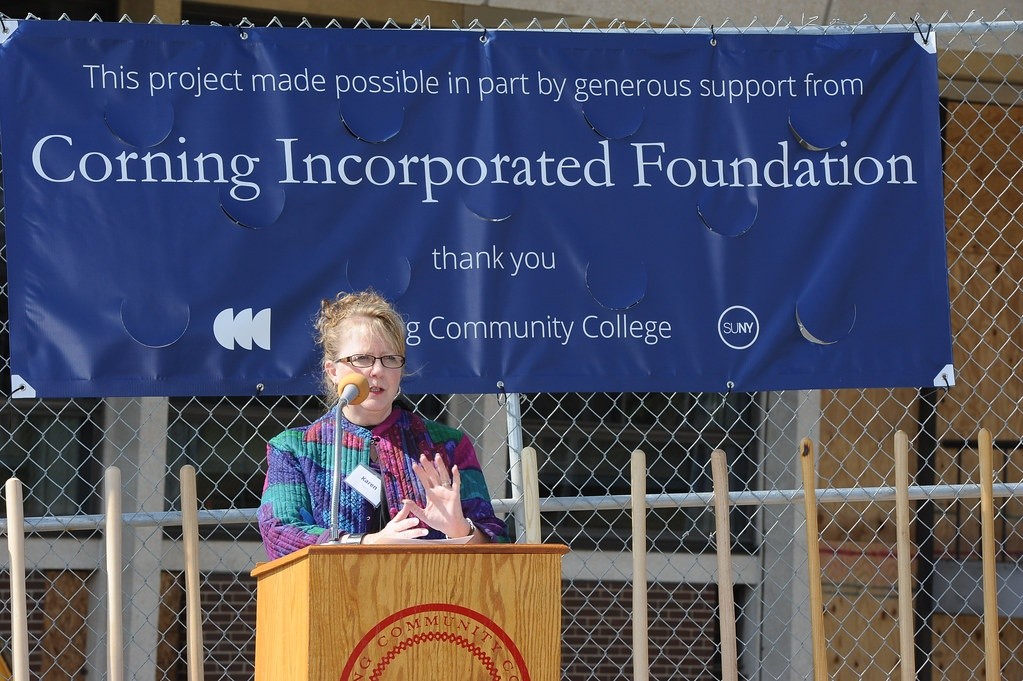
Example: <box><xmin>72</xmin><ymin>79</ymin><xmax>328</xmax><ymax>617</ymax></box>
<box><xmin>257</xmin><ymin>289</ymin><xmax>512</xmax><ymax>561</ymax></box>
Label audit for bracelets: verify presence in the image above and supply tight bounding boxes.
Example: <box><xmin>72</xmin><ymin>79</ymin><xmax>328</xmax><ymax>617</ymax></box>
<box><xmin>446</xmin><ymin>517</ymin><xmax>475</xmax><ymax>539</ymax></box>
<box><xmin>346</xmin><ymin>532</ymin><xmax>366</xmax><ymax>545</ymax></box>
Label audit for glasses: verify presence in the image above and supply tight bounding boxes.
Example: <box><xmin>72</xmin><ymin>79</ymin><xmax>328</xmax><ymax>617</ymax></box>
<box><xmin>335</xmin><ymin>354</ymin><xmax>406</xmax><ymax>369</ymax></box>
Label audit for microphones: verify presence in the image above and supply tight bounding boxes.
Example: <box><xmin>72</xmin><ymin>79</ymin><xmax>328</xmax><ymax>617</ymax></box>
<box><xmin>321</xmin><ymin>373</ymin><xmax>369</xmax><ymax>546</ymax></box>
